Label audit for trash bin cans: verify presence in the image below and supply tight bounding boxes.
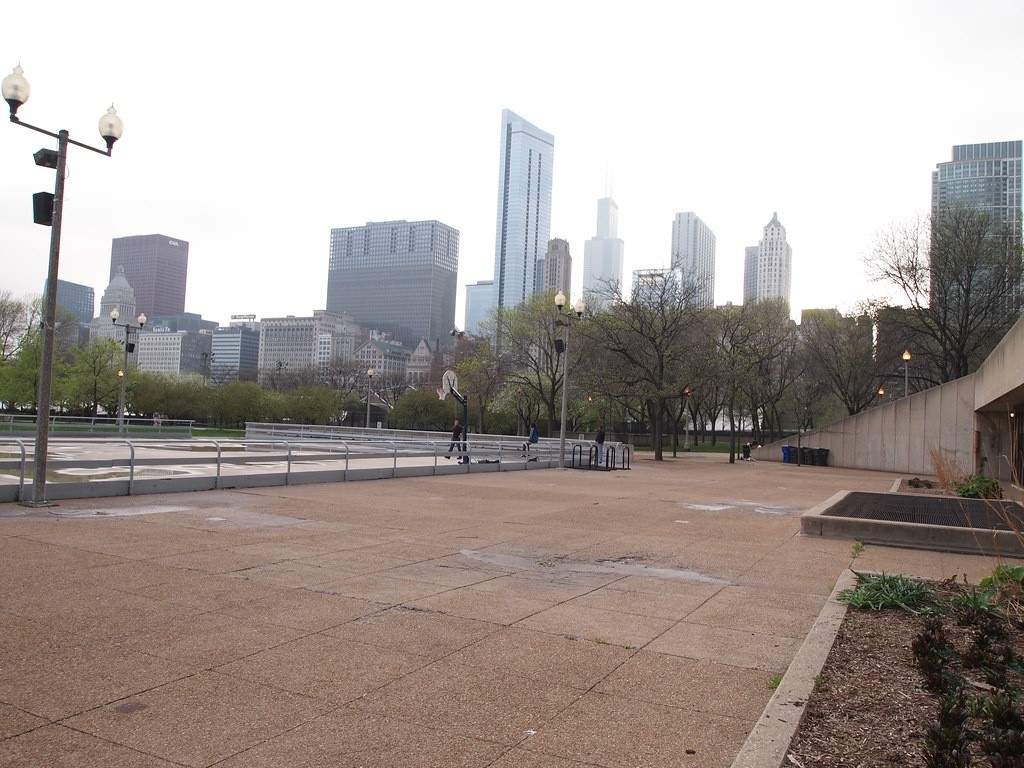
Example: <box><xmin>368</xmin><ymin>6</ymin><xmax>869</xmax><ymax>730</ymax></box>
<box><xmin>781</xmin><ymin>444</ymin><xmax>829</xmax><ymax>466</ymax></box>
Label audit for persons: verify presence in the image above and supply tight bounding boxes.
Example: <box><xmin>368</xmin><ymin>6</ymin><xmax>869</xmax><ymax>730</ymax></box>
<box><xmin>519</xmin><ymin>422</ymin><xmax>538</xmax><ymax>457</ymax></box>
<box><xmin>595</xmin><ymin>425</ymin><xmax>605</xmax><ymax>463</ymax></box>
<box><xmin>443</xmin><ymin>418</ymin><xmax>463</xmax><ymax>459</ymax></box>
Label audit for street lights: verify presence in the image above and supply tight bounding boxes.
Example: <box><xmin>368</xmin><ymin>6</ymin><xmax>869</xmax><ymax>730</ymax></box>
<box><xmin>276</xmin><ymin>360</ymin><xmax>288</xmax><ymax>391</ymax></box>
<box><xmin>111</xmin><ymin>308</ymin><xmax>147</xmax><ymax>433</ymax></box>
<box><xmin>2</xmin><ymin>63</ymin><xmax>125</xmax><ymax>508</ymax></box>
<box><xmin>199</xmin><ymin>351</ymin><xmax>215</xmax><ymax>387</ymax></box>
<box><xmin>555</xmin><ymin>289</ymin><xmax>584</xmax><ymax>472</ymax></box>
<box><xmin>367</xmin><ymin>369</ymin><xmax>374</xmax><ymax>428</ymax></box>
<box><xmin>878</xmin><ymin>388</ymin><xmax>884</xmax><ymax>405</ymax></box>
<box><xmin>683</xmin><ymin>388</ymin><xmax>691</xmax><ymax>450</ymax></box>
<box><xmin>903</xmin><ymin>350</ymin><xmax>911</xmax><ymax>397</ymax></box>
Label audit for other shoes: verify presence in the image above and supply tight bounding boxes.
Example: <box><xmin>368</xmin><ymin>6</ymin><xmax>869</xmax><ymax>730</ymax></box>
<box><xmin>457</xmin><ymin>457</ymin><xmax>462</xmax><ymax>459</ymax></box>
<box><xmin>445</xmin><ymin>456</ymin><xmax>450</xmax><ymax>459</ymax></box>
